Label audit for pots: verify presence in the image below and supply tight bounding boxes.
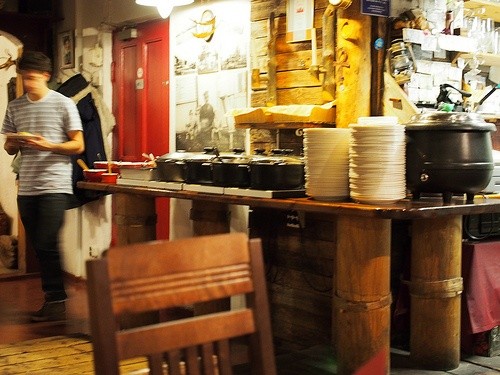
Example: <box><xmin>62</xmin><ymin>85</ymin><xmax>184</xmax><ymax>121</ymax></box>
<box><xmin>212</xmin><ymin>147</ymin><xmax>252</xmax><ymax>186</ymax></box>
<box><xmin>154</xmin><ymin>149</ymin><xmax>185</xmax><ymax>181</ymax></box>
<box><xmin>406</xmin><ymin>114</ymin><xmax>495</xmax><ymax>202</ymax></box>
<box><xmin>181</xmin><ymin>147</ymin><xmax>218</xmax><ymax>184</ymax></box>
<box><xmin>251</xmin><ymin>146</ymin><xmax>304</xmax><ymax>188</ymax></box>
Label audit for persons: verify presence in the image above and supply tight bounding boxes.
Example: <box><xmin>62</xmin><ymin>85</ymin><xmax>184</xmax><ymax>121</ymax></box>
<box><xmin>0</xmin><ymin>51</ymin><xmax>85</xmax><ymax>321</ymax></box>
<box><xmin>199</xmin><ymin>90</ymin><xmax>216</xmax><ymax>140</ymax></box>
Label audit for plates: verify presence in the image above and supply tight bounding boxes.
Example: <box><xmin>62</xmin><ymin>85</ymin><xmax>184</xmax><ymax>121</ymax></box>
<box><xmin>349</xmin><ymin>124</ymin><xmax>407</xmax><ymax>205</ymax></box>
<box><xmin>303</xmin><ymin>127</ymin><xmax>349</xmax><ymax>200</ymax></box>
<box><xmin>5</xmin><ymin>134</ymin><xmax>42</xmax><ymax>139</ymax></box>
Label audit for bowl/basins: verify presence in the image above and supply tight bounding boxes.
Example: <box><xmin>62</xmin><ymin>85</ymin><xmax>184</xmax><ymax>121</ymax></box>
<box><xmin>83</xmin><ymin>169</ymin><xmax>118</xmax><ymax>183</ymax></box>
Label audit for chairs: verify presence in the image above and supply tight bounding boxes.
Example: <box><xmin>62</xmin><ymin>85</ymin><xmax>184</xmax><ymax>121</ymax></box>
<box><xmin>85</xmin><ymin>232</ymin><xmax>276</xmax><ymax>375</ymax></box>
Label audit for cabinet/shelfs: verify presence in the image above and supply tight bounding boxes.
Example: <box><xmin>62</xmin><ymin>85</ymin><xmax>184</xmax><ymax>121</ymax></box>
<box><xmin>384</xmin><ymin>27</ymin><xmax>473</xmax><ymax>124</ymax></box>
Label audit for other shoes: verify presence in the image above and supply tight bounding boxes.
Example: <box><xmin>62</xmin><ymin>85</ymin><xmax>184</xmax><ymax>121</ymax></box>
<box><xmin>31</xmin><ymin>298</ymin><xmax>68</xmax><ymax>327</ymax></box>
